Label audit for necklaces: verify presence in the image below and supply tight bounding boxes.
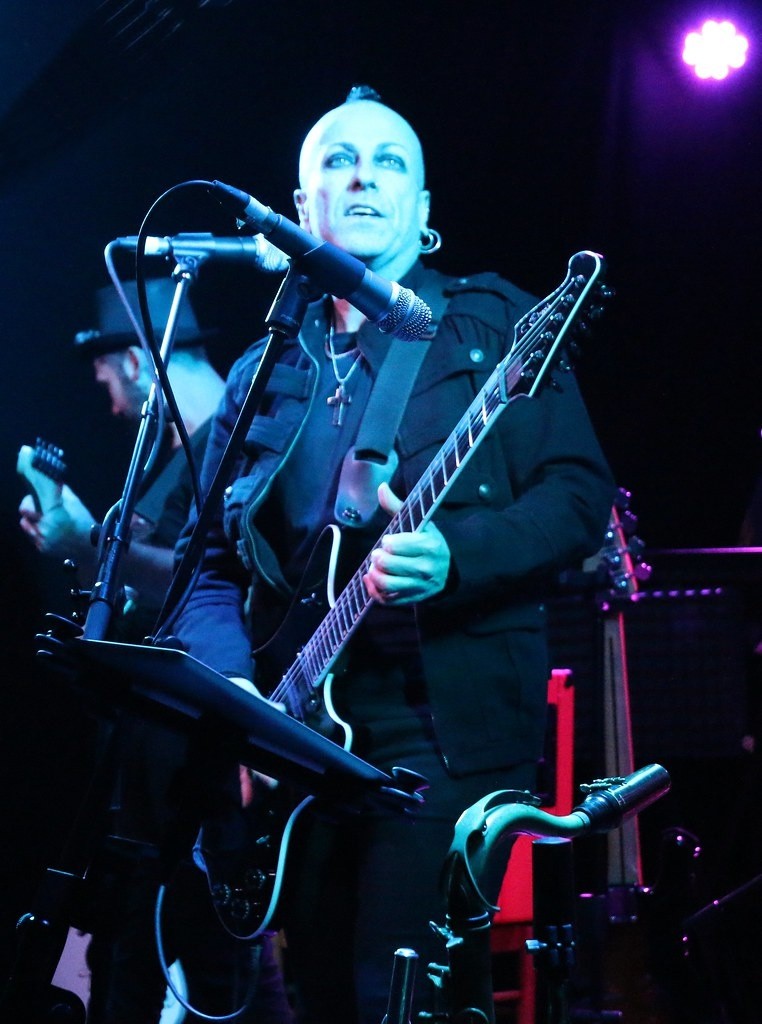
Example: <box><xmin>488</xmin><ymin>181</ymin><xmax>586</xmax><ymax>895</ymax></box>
<box><xmin>323</xmin><ymin>318</ymin><xmax>364</xmax><ymax>428</ymax></box>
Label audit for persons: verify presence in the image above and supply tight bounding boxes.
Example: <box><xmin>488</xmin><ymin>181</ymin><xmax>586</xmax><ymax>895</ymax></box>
<box><xmin>20</xmin><ymin>82</ymin><xmax>616</xmax><ymax>1024</ymax></box>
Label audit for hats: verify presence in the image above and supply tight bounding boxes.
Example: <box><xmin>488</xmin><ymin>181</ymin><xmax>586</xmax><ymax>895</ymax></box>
<box><xmin>59</xmin><ymin>276</ymin><xmax>216</xmax><ymax>392</ymax></box>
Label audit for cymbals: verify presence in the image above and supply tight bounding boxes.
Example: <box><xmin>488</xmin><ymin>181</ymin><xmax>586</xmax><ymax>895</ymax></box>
<box><xmin>652</xmin><ymin>543</ymin><xmax>760</xmax><ymax>557</ymax></box>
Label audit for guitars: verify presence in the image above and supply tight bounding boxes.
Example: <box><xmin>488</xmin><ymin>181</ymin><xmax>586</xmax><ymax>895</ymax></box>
<box><xmin>201</xmin><ymin>250</ymin><xmax>612</xmax><ymax>944</ymax></box>
<box><xmin>13</xmin><ymin>435</ymin><xmax>100</xmax><ymax>589</ymax></box>
<box><xmin>561</xmin><ymin>484</ymin><xmax>735</xmax><ymax>1023</ymax></box>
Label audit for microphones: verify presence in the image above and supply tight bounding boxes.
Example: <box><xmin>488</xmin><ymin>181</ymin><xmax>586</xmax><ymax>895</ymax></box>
<box><xmin>207</xmin><ymin>180</ymin><xmax>432</xmax><ymax>343</ymax></box>
<box><xmin>113</xmin><ymin>229</ymin><xmax>291</xmax><ymax>288</ymax></box>
